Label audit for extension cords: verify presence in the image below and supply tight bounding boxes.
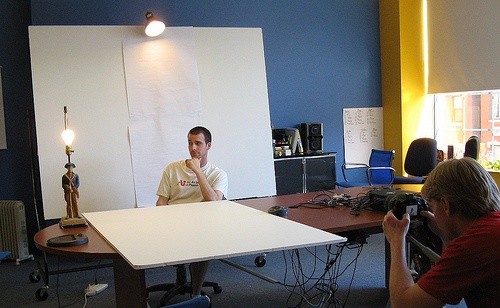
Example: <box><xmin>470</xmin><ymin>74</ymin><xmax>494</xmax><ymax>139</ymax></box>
<box><xmin>84</xmin><ymin>283</ymin><xmax>109</xmax><ymax>297</ymax></box>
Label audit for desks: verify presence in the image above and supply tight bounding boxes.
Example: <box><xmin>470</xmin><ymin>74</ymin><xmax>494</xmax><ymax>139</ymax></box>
<box><xmin>33</xmin><ymin>184</ymin><xmax>432</xmax><ymax>308</ymax></box>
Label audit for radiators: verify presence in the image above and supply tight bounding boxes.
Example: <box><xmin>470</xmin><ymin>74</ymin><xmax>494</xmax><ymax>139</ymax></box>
<box><xmin>0</xmin><ymin>199</ymin><xmax>35</xmax><ymax>265</ymax></box>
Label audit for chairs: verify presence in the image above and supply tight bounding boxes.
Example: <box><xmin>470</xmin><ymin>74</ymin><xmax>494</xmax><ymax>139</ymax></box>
<box><xmin>146</xmin><ymin>264</ymin><xmax>222</xmax><ymax>307</ymax></box>
<box><xmin>342</xmin><ymin>135</ymin><xmax>480</xmax><ymax>185</ymax></box>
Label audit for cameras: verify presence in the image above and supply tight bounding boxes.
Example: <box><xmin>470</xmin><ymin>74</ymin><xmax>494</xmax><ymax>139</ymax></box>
<box><xmin>383</xmin><ymin>193</ymin><xmax>428</xmax><ymax>221</ymax></box>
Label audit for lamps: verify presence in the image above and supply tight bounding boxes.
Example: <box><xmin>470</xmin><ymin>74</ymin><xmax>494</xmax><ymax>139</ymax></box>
<box><xmin>144</xmin><ymin>10</ymin><xmax>165</xmax><ymax>38</ymax></box>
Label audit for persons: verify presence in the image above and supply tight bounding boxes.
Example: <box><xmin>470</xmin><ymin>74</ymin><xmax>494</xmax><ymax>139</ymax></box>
<box><xmin>156</xmin><ymin>126</ymin><xmax>228</xmax><ymax>296</ymax></box>
<box><xmin>62</xmin><ymin>163</ymin><xmax>80</xmax><ymax>218</ymax></box>
<box><xmin>382</xmin><ymin>156</ymin><xmax>500</xmax><ymax>308</ymax></box>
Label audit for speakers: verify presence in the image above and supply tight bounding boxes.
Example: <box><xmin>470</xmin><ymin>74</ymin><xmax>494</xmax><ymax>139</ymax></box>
<box><xmin>301</xmin><ymin>122</ymin><xmax>324</xmax><ymax>155</ymax></box>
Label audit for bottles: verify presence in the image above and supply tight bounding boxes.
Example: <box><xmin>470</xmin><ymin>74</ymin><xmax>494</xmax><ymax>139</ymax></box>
<box><xmin>272</xmin><ymin>139</ymin><xmax>276</xmax><ymax>156</ymax></box>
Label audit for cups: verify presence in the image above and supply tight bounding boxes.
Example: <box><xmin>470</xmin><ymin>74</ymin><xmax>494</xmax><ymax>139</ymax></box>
<box><xmin>275</xmin><ymin>146</ymin><xmax>291</xmax><ymax>157</ymax></box>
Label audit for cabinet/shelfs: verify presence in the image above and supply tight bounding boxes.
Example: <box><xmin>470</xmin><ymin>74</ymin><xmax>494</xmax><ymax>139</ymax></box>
<box><xmin>274</xmin><ymin>151</ymin><xmax>337</xmax><ymax>196</ymax></box>
